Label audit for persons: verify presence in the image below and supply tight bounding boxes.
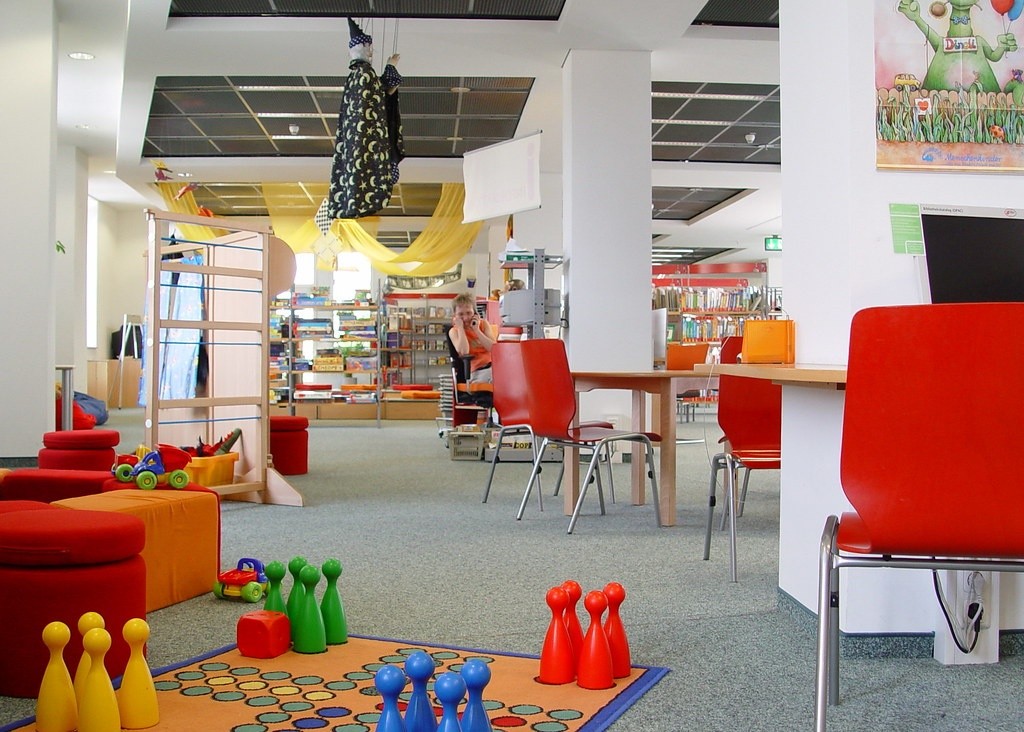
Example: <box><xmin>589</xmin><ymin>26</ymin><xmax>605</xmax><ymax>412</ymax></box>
<box><xmin>448</xmin><ymin>292</ymin><xmax>500</xmax><ymax>384</ymax></box>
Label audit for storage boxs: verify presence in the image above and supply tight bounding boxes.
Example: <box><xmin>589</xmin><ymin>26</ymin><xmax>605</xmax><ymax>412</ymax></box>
<box><xmin>344</xmin><ymin>357</ymin><xmax>378</xmax><ymax>371</ymax></box>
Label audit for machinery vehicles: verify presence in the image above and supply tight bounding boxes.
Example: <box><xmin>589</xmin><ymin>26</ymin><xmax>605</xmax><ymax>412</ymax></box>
<box><xmin>111</xmin><ymin>444</ymin><xmax>192</xmax><ymax>489</ymax></box>
<box><xmin>213</xmin><ymin>558</ymin><xmax>271</xmax><ymax>602</ymax></box>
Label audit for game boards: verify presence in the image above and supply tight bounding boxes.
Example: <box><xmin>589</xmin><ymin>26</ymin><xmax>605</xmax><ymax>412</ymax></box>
<box><xmin>0</xmin><ymin>633</ymin><xmax>671</xmax><ymax>732</ymax></box>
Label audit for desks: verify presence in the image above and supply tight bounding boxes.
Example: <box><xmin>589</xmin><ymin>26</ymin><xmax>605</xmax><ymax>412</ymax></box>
<box><xmin>565</xmin><ymin>370</ymin><xmax>737</xmax><ymax>527</ymax></box>
<box><xmin>694</xmin><ymin>363</ymin><xmax>848</xmax><ymax>391</ymax></box>
<box><xmin>88</xmin><ymin>359</ymin><xmax>140</xmax><ymax>406</ymax></box>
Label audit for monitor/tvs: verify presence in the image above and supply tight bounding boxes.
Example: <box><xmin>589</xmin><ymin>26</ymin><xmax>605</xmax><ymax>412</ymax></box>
<box><xmin>912</xmin><ymin>202</ymin><xmax>1024</xmax><ymax>304</ymax></box>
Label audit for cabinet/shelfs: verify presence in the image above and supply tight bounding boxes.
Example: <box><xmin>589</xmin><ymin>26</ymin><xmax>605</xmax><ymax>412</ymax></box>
<box><xmin>650</xmin><ymin>256</ymin><xmax>783</xmax><ymax>409</ymax></box>
<box><xmin>495</xmin><ymin>246</ymin><xmax>562</xmax><ymax>340</ymax></box>
<box><xmin>270</xmin><ymin>277</ymin><xmax>458</xmax><ymax>421</ymax></box>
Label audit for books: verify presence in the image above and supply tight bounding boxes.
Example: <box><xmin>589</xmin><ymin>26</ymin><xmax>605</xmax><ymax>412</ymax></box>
<box><xmin>651</xmin><ymin>284</ymin><xmax>784</xmax><ymax>341</ymax></box>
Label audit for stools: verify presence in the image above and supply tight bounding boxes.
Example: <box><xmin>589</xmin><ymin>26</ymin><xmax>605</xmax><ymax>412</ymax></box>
<box><xmin>38</xmin><ymin>429</ymin><xmax>120</xmax><ymax>471</ymax></box>
<box><xmin>270</xmin><ymin>415</ymin><xmax>309</xmax><ymax>476</ymax></box>
<box><xmin>0</xmin><ymin>467</ymin><xmax>220</xmax><ymax>696</ymax></box>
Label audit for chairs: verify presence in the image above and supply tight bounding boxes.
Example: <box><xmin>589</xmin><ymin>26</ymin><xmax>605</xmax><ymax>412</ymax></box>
<box><xmin>442</xmin><ymin>323</ymin><xmax>665</xmax><ymax>534</ymax></box>
<box><xmin>700</xmin><ymin>335</ymin><xmax>781</xmax><ymax>581</ymax></box>
<box><xmin>814</xmin><ymin>303</ymin><xmax>1024</xmax><ymax>732</ymax></box>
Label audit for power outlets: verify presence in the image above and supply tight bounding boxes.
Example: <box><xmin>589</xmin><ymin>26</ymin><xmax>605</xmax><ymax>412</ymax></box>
<box><xmin>184</xmin><ymin>452</ymin><xmax>239</xmax><ymax>502</ymax></box>
<box><xmin>956</xmin><ymin>568</ymin><xmax>993</xmax><ymax>632</ymax></box>
